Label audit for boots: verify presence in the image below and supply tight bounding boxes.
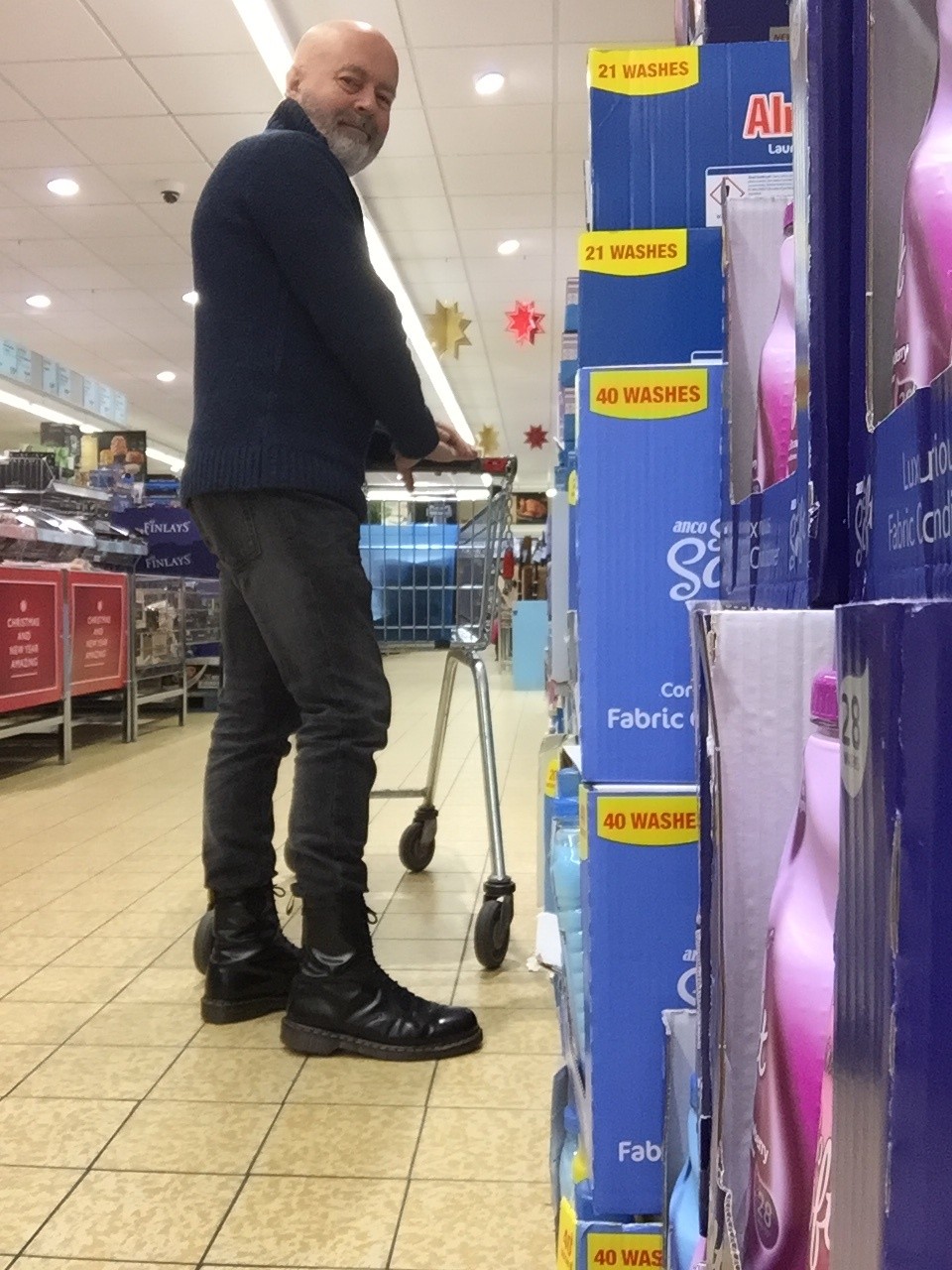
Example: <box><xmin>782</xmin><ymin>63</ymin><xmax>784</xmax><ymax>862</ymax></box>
<box><xmin>201</xmin><ymin>885</ymin><xmax>303</xmax><ymax>1023</ymax></box>
<box><xmin>281</xmin><ymin>891</ymin><xmax>484</xmax><ymax>1059</ymax></box>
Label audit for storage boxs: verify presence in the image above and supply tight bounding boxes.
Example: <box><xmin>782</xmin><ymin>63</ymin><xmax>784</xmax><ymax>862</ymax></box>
<box><xmin>537</xmin><ymin>3</ymin><xmax>952</xmax><ymax>1270</ymax></box>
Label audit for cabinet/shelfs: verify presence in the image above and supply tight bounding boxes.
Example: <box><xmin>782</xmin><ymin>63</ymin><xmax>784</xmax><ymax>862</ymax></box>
<box><xmin>1</xmin><ymin>567</ymin><xmax>223</xmax><ymax>767</ymax></box>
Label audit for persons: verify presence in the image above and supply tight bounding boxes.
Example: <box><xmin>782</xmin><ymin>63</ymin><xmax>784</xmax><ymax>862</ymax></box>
<box><xmin>180</xmin><ymin>15</ymin><xmax>489</xmax><ymax>1065</ymax></box>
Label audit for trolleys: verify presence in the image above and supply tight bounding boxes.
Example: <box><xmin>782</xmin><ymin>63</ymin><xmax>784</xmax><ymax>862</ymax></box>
<box><xmin>357</xmin><ymin>454</ymin><xmax>522</xmax><ymax>971</ymax></box>
